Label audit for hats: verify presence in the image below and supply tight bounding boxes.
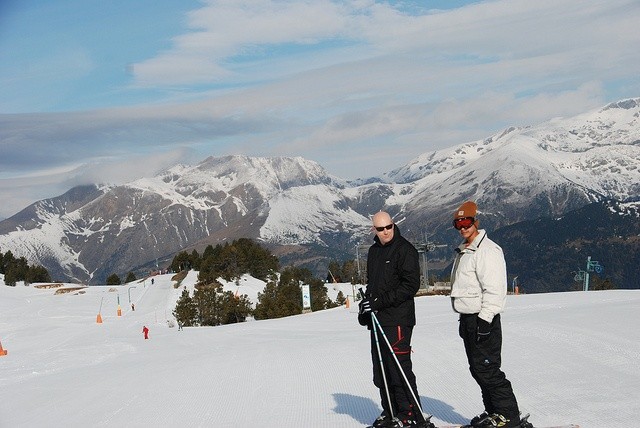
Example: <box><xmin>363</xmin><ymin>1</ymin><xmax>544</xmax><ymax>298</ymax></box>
<box><xmin>455</xmin><ymin>202</ymin><xmax>479</xmax><ymax>228</ymax></box>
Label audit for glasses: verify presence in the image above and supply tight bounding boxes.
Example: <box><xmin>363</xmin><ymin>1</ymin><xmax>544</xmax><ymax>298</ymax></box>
<box><xmin>374</xmin><ymin>224</ymin><xmax>392</xmax><ymax>231</ymax></box>
<box><xmin>452</xmin><ymin>217</ymin><xmax>474</xmax><ymax>230</ymax></box>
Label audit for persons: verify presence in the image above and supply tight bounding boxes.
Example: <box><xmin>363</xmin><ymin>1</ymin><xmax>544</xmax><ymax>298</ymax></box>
<box><xmin>358</xmin><ymin>210</ymin><xmax>423</xmax><ymax>427</ymax></box>
<box><xmin>445</xmin><ymin>200</ymin><xmax>521</xmax><ymax>427</ymax></box>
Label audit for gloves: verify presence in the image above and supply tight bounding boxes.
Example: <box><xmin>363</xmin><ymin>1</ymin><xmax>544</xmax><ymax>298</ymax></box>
<box><xmin>359</xmin><ymin>297</ymin><xmax>385</xmax><ymax>314</ymax></box>
<box><xmin>476</xmin><ymin>317</ymin><xmax>491</xmax><ymax>343</ymax></box>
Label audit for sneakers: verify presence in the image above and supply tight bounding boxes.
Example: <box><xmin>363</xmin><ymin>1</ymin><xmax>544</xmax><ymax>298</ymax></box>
<box><xmin>471</xmin><ymin>411</ymin><xmax>490</xmax><ymax>428</ymax></box>
<box><xmin>389</xmin><ymin>411</ymin><xmax>435</xmax><ymax>428</ymax></box>
<box><xmin>373</xmin><ymin>410</ymin><xmax>397</xmax><ymax>428</ymax></box>
<box><xmin>477</xmin><ymin>412</ymin><xmax>524</xmax><ymax>428</ymax></box>
